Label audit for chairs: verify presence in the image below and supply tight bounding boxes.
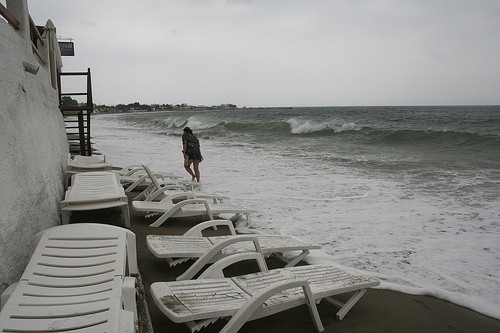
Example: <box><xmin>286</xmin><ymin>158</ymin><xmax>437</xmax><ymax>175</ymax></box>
<box><xmin>59</xmin><ymin>171</ymin><xmax>131</xmax><ymax>229</ymax></box>
<box><xmin>65</xmin><ymin>152</ymin><xmax>113</xmax><ymax>193</ymax></box>
<box><xmin>115</xmin><ymin>163</ymin><xmax>260</xmax><ymax>230</ymax></box>
<box><xmin>149</xmin><ymin>251</ymin><xmax>380</xmax><ymax>333</ymax></box>
<box><xmin>0</xmin><ymin>223</ymin><xmax>139</xmax><ymax>333</ymax></box>
<box><xmin>146</xmin><ymin>220</ymin><xmax>322</xmax><ymax>281</ymax></box>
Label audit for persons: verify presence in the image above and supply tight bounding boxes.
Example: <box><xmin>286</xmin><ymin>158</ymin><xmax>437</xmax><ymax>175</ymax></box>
<box><xmin>182</xmin><ymin>127</ymin><xmax>203</xmax><ymax>183</ymax></box>
